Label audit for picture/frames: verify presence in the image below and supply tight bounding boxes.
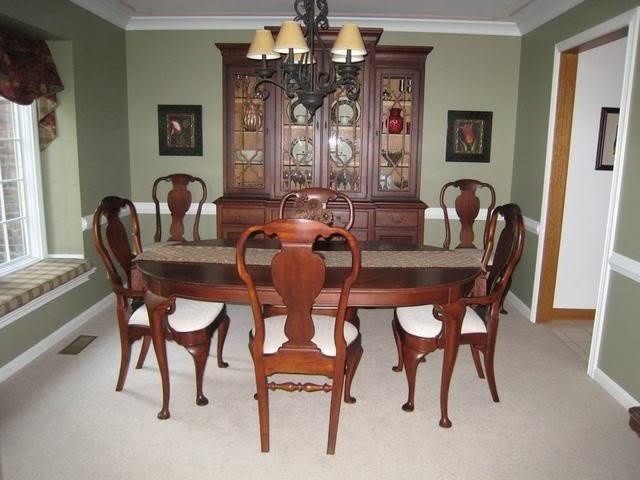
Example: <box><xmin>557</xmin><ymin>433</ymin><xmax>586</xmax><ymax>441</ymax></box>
<box><xmin>445</xmin><ymin>110</ymin><xmax>493</xmax><ymax>164</ymax></box>
<box><xmin>593</xmin><ymin>106</ymin><xmax>619</xmax><ymax>172</ymax></box>
<box><xmin>155</xmin><ymin>102</ymin><xmax>205</xmax><ymax>160</ymax></box>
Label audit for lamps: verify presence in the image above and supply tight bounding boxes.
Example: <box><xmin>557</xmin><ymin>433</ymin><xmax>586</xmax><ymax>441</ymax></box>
<box><xmin>246</xmin><ymin>0</ymin><xmax>369</xmax><ymax>124</ymax></box>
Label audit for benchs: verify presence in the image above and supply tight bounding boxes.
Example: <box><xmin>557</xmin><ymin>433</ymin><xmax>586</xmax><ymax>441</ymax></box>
<box><xmin>1</xmin><ymin>256</ymin><xmax>91</xmax><ymax>367</ymax></box>
